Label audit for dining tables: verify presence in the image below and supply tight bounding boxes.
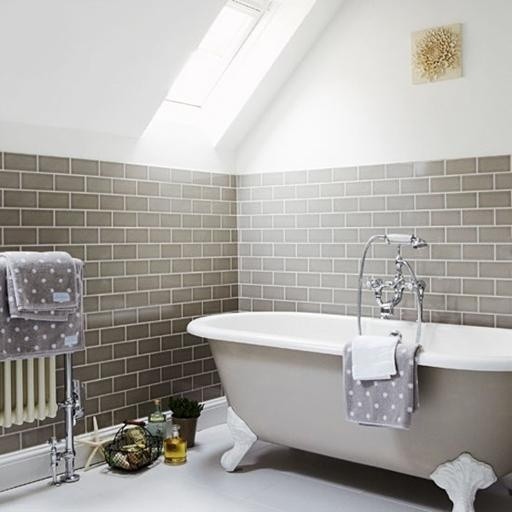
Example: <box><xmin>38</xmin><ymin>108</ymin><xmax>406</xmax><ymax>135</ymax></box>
<box><xmin>168</xmin><ymin>396</ymin><xmax>205</xmax><ymax>448</ymax></box>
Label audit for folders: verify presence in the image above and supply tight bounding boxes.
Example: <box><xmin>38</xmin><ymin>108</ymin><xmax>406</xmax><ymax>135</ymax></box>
<box><xmin>0</xmin><ymin>354</ymin><xmax>59</xmax><ymax>429</ymax></box>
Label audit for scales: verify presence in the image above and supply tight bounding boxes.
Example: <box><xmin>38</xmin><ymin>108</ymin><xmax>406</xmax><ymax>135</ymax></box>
<box><xmin>186</xmin><ymin>310</ymin><xmax>512</xmax><ymax>481</ymax></box>
